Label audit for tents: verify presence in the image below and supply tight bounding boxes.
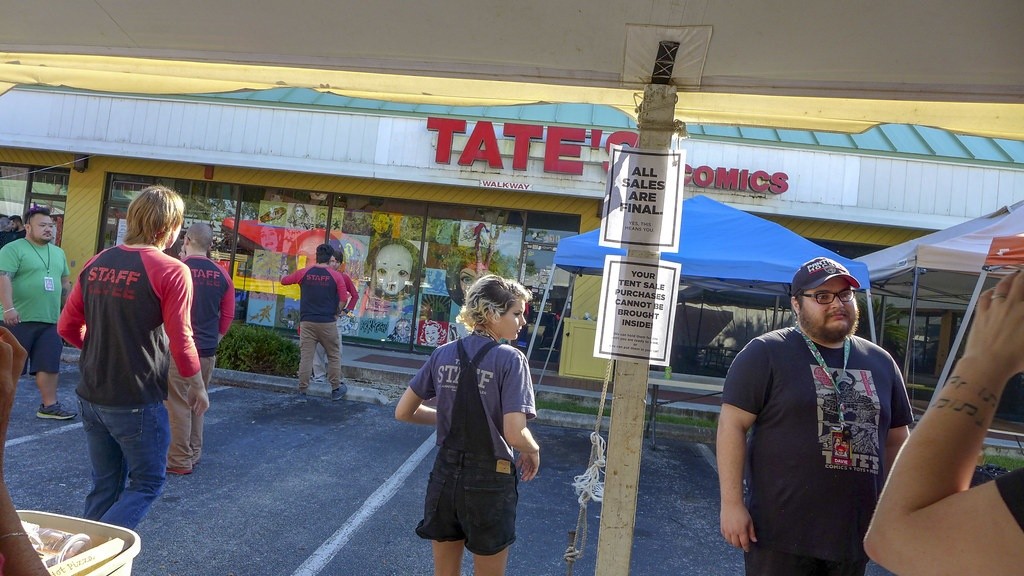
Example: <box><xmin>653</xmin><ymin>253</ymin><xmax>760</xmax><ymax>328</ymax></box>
<box><xmin>856</xmin><ymin>200</ymin><xmax>1024</xmax><ymax>401</ymax></box>
<box><xmin>526</xmin><ymin>195</ymin><xmax>876</xmax><ymax>397</ymax></box>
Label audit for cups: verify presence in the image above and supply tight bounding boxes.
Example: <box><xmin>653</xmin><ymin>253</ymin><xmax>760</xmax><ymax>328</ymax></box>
<box><xmin>31</xmin><ymin>528</ymin><xmax>93</xmax><ymax>564</ymax></box>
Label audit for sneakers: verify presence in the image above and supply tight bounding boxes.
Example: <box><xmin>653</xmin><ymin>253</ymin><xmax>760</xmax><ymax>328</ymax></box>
<box><xmin>37</xmin><ymin>401</ymin><xmax>77</xmax><ymax>420</ymax></box>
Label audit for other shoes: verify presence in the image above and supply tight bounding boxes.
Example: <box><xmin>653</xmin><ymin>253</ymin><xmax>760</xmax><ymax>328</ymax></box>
<box><xmin>331</xmin><ymin>382</ymin><xmax>347</xmax><ymax>401</ymax></box>
<box><xmin>296</xmin><ymin>392</ymin><xmax>308</xmax><ymax>403</ymax></box>
<box><xmin>166</xmin><ymin>468</ymin><xmax>192</xmax><ymax>474</ymax></box>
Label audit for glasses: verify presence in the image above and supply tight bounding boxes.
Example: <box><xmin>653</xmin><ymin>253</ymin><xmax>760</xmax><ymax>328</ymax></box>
<box><xmin>799</xmin><ymin>289</ymin><xmax>855</xmax><ymax>304</ymax></box>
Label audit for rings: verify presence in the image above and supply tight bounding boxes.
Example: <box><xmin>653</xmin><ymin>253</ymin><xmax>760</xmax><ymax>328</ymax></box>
<box><xmin>991</xmin><ymin>294</ymin><xmax>1006</xmax><ymax>299</ymax></box>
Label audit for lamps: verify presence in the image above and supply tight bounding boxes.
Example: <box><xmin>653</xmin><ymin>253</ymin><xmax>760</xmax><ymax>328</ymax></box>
<box><xmin>73</xmin><ymin>155</ymin><xmax>88</xmax><ymax>173</ymax></box>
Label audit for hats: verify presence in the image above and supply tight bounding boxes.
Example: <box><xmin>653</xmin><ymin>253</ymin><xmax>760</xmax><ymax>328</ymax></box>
<box><xmin>791</xmin><ymin>257</ymin><xmax>861</xmax><ymax>299</ymax></box>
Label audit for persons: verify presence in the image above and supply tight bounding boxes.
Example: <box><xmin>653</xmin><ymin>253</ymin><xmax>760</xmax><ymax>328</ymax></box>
<box><xmin>313</xmin><ymin>250</ymin><xmax>359</xmax><ymax>382</ymax></box>
<box><xmin>7</xmin><ymin>215</ymin><xmax>26</xmax><ymax>243</ymax></box>
<box><xmin>0</xmin><ymin>206</ymin><xmax>77</xmax><ymax>419</ymax></box>
<box><xmin>0</xmin><ymin>326</ymin><xmax>50</xmax><ymax>576</ymax></box>
<box><xmin>395</xmin><ymin>275</ymin><xmax>540</xmax><ymax>576</ymax></box>
<box><xmin>863</xmin><ymin>268</ymin><xmax>1024</xmax><ymax>576</ymax></box>
<box><xmin>165</xmin><ymin>223</ymin><xmax>235</xmax><ymax>474</ymax></box>
<box><xmin>281</xmin><ymin>244</ymin><xmax>347</xmax><ymax>402</ymax></box>
<box><xmin>57</xmin><ymin>185</ymin><xmax>210</xmax><ymax>532</ymax></box>
<box><xmin>717</xmin><ymin>258</ymin><xmax>913</xmax><ymax>576</ymax></box>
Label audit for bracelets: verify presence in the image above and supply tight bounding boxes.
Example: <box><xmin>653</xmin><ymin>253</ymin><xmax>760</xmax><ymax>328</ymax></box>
<box><xmin>283</xmin><ymin>269</ymin><xmax>288</xmax><ymax>272</ymax></box>
<box><xmin>4</xmin><ymin>307</ymin><xmax>14</xmax><ymax>313</ymax></box>
<box><xmin>0</xmin><ymin>533</ymin><xmax>28</xmax><ymax>539</ymax></box>
<box><xmin>343</xmin><ymin>309</ymin><xmax>348</xmax><ymax>313</ymax></box>
